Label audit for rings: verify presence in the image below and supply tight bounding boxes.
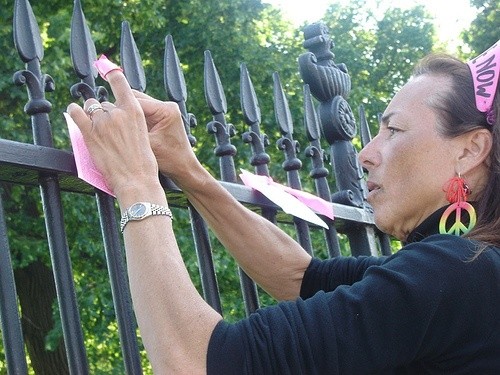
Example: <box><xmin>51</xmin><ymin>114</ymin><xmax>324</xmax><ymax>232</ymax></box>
<box><xmin>86</xmin><ymin>103</ymin><xmax>103</xmax><ymax>119</ymax></box>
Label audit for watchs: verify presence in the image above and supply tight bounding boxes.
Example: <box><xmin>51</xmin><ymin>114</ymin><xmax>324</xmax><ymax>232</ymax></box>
<box><xmin>118</xmin><ymin>202</ymin><xmax>173</xmax><ymax>232</ymax></box>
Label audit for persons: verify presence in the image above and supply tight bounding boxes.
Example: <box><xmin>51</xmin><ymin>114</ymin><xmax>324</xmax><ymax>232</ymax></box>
<box><xmin>66</xmin><ymin>40</ymin><xmax>500</xmax><ymax>375</ymax></box>
